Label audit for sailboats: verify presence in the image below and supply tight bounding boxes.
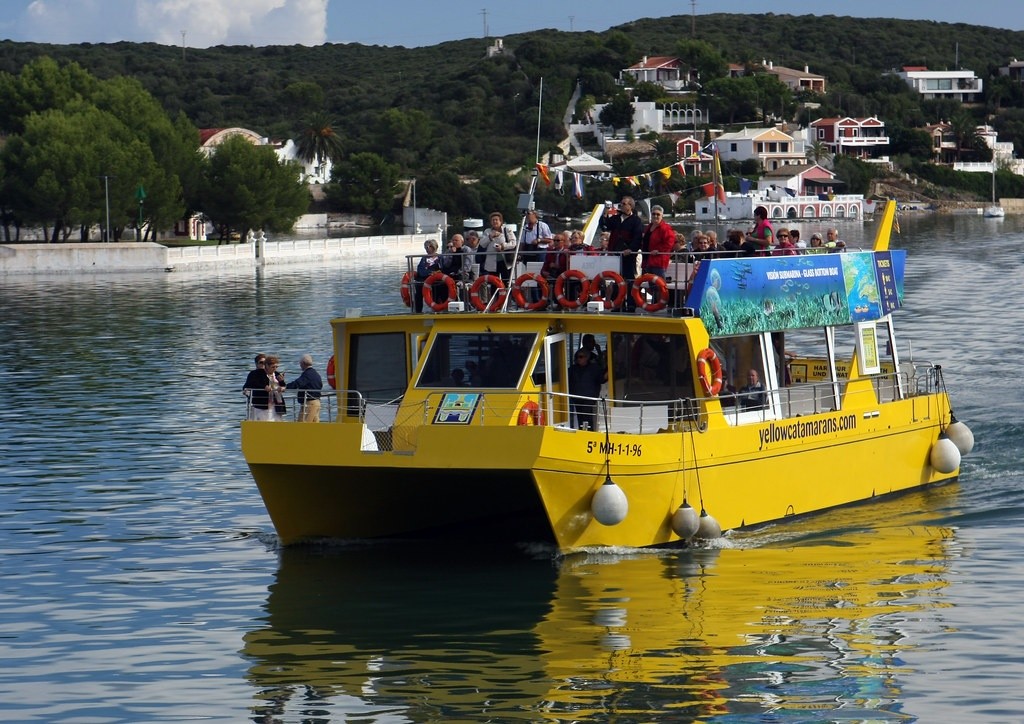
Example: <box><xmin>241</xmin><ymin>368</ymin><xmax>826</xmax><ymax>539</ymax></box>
<box><xmin>982</xmin><ymin>141</ymin><xmax>1006</xmax><ymax>217</ymax></box>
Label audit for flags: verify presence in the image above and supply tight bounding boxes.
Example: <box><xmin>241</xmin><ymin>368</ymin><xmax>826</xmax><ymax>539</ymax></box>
<box><xmin>537</xmin><ymin>143</ymin><xmax>716</xmax><ymax>199</ymax></box>
<box><xmin>714</xmin><ymin>144</ymin><xmax>727</xmax><ymax>206</ymax></box>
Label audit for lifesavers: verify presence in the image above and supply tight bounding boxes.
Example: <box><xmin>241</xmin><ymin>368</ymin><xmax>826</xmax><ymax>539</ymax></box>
<box><xmin>422</xmin><ymin>272</ymin><xmax>458</xmax><ymax>312</ymax></box>
<box><xmin>697</xmin><ymin>349</ymin><xmax>723</xmax><ymax>396</ymax></box>
<box><xmin>589</xmin><ymin>270</ymin><xmax>627</xmax><ymax>309</ymax></box>
<box><xmin>518</xmin><ymin>400</ymin><xmax>545</xmax><ymax>426</ymax></box>
<box><xmin>470</xmin><ymin>275</ymin><xmax>507</xmax><ymax>312</ymax></box>
<box><xmin>401</xmin><ymin>271</ymin><xmax>417</xmax><ymax>307</ymax></box>
<box><xmin>554</xmin><ymin>270</ymin><xmax>589</xmax><ymax>309</ymax></box>
<box><xmin>631</xmin><ymin>273</ymin><xmax>669</xmax><ymax>312</ymax></box>
<box><xmin>326</xmin><ymin>355</ymin><xmax>336</xmax><ymax>389</ymax></box>
<box><xmin>512</xmin><ymin>273</ymin><xmax>550</xmax><ymax>310</ymax></box>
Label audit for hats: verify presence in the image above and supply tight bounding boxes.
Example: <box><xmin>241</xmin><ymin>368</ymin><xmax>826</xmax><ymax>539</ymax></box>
<box><xmin>722</xmin><ymin>370</ymin><xmax>728</xmax><ymax>376</ymax></box>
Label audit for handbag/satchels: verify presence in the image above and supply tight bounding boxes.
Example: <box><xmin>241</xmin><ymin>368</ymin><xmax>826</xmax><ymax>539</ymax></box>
<box><xmin>504</xmin><ymin>227</ymin><xmax>516</xmax><ymax>265</ymax></box>
<box><xmin>273</xmin><ymin>396</ymin><xmax>287</xmax><ymax>415</ymax></box>
<box><xmin>522</xmin><ymin>243</ymin><xmax>538</xmax><ymax>257</ymax></box>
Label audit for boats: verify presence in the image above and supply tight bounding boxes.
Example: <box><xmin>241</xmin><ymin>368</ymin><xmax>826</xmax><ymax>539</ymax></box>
<box><xmin>240</xmin><ymin>477</ymin><xmax>953</xmax><ymax>723</ymax></box>
<box><xmin>239</xmin><ymin>195</ymin><xmax>976</xmax><ymax>552</ymax></box>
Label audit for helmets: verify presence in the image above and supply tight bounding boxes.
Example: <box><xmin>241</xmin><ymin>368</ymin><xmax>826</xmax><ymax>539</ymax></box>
<box><xmin>811</xmin><ymin>233</ymin><xmax>824</xmax><ymax>246</ymax></box>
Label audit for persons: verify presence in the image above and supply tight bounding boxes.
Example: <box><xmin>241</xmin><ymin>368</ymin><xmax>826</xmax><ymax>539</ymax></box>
<box><xmin>449</xmin><ymin>367</ymin><xmax>465</xmax><ymax>386</ymax></box>
<box><xmin>280</xmin><ymin>352</ymin><xmax>323</xmax><ymax>424</ymax></box>
<box><xmin>784</xmin><ymin>348</ymin><xmax>799</xmax><ymax>384</ymax></box>
<box><xmin>600</xmin><ymin>197</ymin><xmax>644</xmax><ymax>313</ymax></box>
<box><xmin>465</xmin><ymin>360</ymin><xmax>478</xmax><ymax>380</ymax></box>
<box><xmin>718</xmin><ymin>369</ymin><xmax>736</xmax><ymax>407</ymax></box>
<box><xmin>514</xmin><ymin>208</ymin><xmax>552</xmax><ymax>305</ymax></box>
<box><xmin>575</xmin><ymin>335</ymin><xmax>608</xmax><ymax>367</ymax></box>
<box><xmin>640</xmin><ymin>205</ymin><xmax>676</xmax><ymax>309</ymax></box>
<box><xmin>244</xmin><ymin>354</ymin><xmax>287</xmax><ymax>421</ymax></box>
<box><xmin>482</xmin><ymin>209</ymin><xmax>517</xmax><ymax>297</ymax></box>
<box><xmin>738</xmin><ymin>369</ymin><xmax>765</xmax><ymax>408</ymax></box>
<box><xmin>415</xmin><ymin>207</ymin><xmax>847</xmax><ymax>315</ymax></box>
<box><xmin>568</xmin><ymin>347</ymin><xmax>608</xmax><ymax>431</ymax></box>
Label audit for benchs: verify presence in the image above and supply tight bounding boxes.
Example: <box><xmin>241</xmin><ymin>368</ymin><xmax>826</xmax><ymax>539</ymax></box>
<box><xmin>462</xmin><ymin>256</ymin><xmax>694</xmax><ymax>303</ymax></box>
<box><xmin>721</xmin><ymin>376</ymin><xmax>900</xmax><ymax>424</ymax></box>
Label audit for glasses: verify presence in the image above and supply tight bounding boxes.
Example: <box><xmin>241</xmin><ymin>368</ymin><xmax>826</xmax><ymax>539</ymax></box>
<box><xmin>652</xmin><ymin>212</ymin><xmax>663</xmax><ymax>216</ymax></box>
<box><xmin>447</xmin><ymin>245</ymin><xmax>454</xmax><ymax>249</ymax></box>
<box><xmin>426</xmin><ymin>245</ymin><xmax>433</xmax><ymax>249</ymax></box>
<box><xmin>270</xmin><ymin>365</ymin><xmax>279</xmax><ymax>368</ymax></box>
<box><xmin>257</xmin><ymin>361</ymin><xmax>266</xmax><ymax>365</ymax></box>
<box><xmin>553</xmin><ymin>239</ymin><xmax>563</xmax><ymax>242</ymax></box>
<box><xmin>777</xmin><ymin>235</ymin><xmax>788</xmax><ymax>237</ymax></box>
<box><xmin>698</xmin><ymin>242</ymin><xmax>709</xmax><ymax>244</ymax></box>
<box><xmin>578</xmin><ymin>354</ymin><xmax>587</xmax><ymax>358</ymax></box>
<box><xmin>466</xmin><ymin>237</ymin><xmax>471</xmax><ymax>241</ymax></box>
<box><xmin>722</xmin><ymin>376</ymin><xmax>729</xmax><ymax>380</ymax></box>
<box><xmin>621</xmin><ymin>202</ymin><xmax>630</xmax><ymax>205</ymax></box>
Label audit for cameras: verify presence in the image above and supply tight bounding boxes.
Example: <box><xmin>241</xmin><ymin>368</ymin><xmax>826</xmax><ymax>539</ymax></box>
<box><xmin>605</xmin><ymin>201</ymin><xmax>613</xmax><ymax>209</ymax></box>
